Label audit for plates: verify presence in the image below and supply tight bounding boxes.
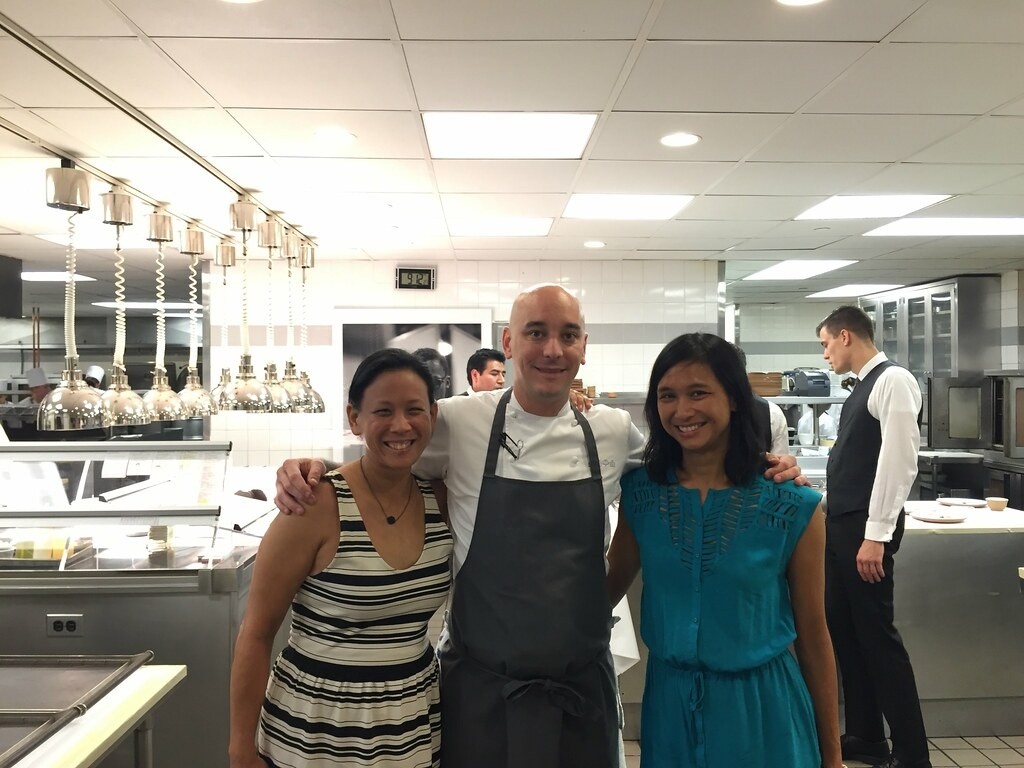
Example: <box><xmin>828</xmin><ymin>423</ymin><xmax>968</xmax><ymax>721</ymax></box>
<box><xmin>909</xmin><ymin>507</ymin><xmax>969</xmax><ymax>523</ymax></box>
<box><xmin>904</xmin><ymin>500</ymin><xmax>940</xmax><ymax>514</ymax></box>
<box><xmin>937</xmin><ymin>497</ymin><xmax>987</xmax><ymax>508</ymax></box>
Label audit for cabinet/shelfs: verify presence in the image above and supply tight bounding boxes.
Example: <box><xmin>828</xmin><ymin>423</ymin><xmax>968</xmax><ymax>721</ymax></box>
<box><xmin>858</xmin><ymin>275</ymin><xmax>1001</xmax><ymax>378</ymax></box>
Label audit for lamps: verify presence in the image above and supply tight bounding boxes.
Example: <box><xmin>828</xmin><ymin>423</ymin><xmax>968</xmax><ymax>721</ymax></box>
<box><xmin>37</xmin><ymin>159</ymin><xmax>324</xmax><ymax>431</ymax></box>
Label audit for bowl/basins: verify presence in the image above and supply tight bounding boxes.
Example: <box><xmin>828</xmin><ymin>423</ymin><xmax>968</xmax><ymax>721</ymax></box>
<box><xmin>986</xmin><ymin>497</ymin><xmax>1010</xmax><ymax>511</ymax></box>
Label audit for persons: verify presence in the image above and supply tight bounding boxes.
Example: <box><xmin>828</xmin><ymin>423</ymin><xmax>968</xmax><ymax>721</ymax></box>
<box><xmin>729</xmin><ymin>341</ymin><xmax>789</xmax><ymax>454</ymax></box>
<box><xmin>828</xmin><ymin>373</ymin><xmax>857</xmax><ymax>424</ymax></box>
<box><xmin>84</xmin><ymin>365</ymin><xmax>105</xmax><ymax>395</ymax></box>
<box><xmin>816</xmin><ymin>305</ymin><xmax>931</xmax><ymax>768</ymax></box>
<box><xmin>0</xmin><ymin>380</ymin><xmax>16</xmax><ymax>428</ymax></box>
<box><xmin>608</xmin><ymin>333</ymin><xmax>846</xmax><ymax>768</ymax></box>
<box><xmin>798</xmin><ymin>370</ymin><xmax>836</xmax><ymax>455</ymax></box>
<box><xmin>459</xmin><ymin>348</ymin><xmax>506</xmax><ymax>396</ymax></box>
<box><xmin>19</xmin><ymin>368</ymin><xmax>48</xmax><ymax>430</ymax></box>
<box><xmin>228</xmin><ymin>349</ymin><xmax>594</xmax><ymax>768</ymax></box>
<box><xmin>412</xmin><ymin>348</ymin><xmax>450</xmax><ymax>401</ymax></box>
<box><xmin>274</xmin><ymin>286</ymin><xmax>812</xmax><ymax>768</ymax></box>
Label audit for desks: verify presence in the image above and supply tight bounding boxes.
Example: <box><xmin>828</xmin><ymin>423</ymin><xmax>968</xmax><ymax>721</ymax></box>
<box><xmin>820</xmin><ymin>499</ymin><xmax>1024</xmax><ymax>736</ymax></box>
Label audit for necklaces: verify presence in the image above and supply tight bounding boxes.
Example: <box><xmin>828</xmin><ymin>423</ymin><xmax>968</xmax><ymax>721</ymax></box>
<box><xmin>360</xmin><ymin>455</ymin><xmax>413</xmax><ymax>524</ymax></box>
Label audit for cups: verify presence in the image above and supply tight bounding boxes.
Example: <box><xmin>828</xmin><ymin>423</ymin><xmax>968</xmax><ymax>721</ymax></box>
<box><xmin>588</xmin><ymin>386</ymin><xmax>595</xmax><ymax>398</ymax></box>
